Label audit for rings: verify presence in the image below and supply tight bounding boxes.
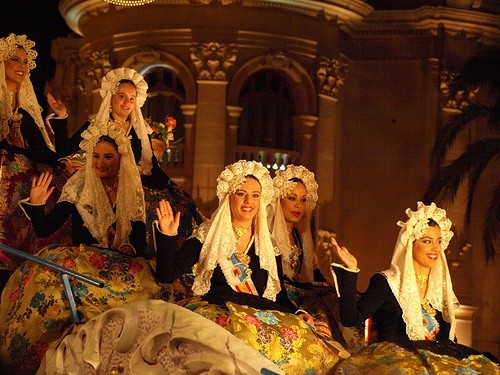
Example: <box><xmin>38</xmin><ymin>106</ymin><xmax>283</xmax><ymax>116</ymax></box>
<box><xmin>161</xmin><ymin>213</ymin><xmax>166</xmax><ymax>217</ymax></box>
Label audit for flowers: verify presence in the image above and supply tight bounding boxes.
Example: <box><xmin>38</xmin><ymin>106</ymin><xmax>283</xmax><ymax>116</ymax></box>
<box><xmin>146</xmin><ymin>115</ymin><xmax>177</xmax><ymax>142</ymax></box>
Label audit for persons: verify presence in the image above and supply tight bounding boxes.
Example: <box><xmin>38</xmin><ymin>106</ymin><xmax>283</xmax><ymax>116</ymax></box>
<box><xmin>152</xmin><ymin>160</ymin><xmax>349</xmax><ymax>351</ymax></box>
<box><xmin>329</xmin><ymin>201</ymin><xmax>500</xmax><ymax>364</ymax></box>
<box><xmin>18</xmin><ymin>120</ymin><xmax>148</xmax><ymax>256</ymax></box>
<box><xmin>0</xmin><ymin>32</ymin><xmax>56</xmax><ymax>162</ymax></box>
<box><xmin>266</xmin><ymin>164</ymin><xmax>337</xmax><ymax>296</ymax></box>
<box><xmin>46</xmin><ymin>66</ymin><xmax>207</xmax><ymax>237</ymax></box>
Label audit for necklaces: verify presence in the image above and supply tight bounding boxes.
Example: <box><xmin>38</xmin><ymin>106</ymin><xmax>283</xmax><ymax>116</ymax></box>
<box><xmin>109</xmin><ymin>111</ymin><xmax>129</xmax><ymax>126</ymax></box>
<box><xmin>232</xmin><ymin>224</ymin><xmax>253</xmax><ymax>246</ymax></box>
<box><xmin>10</xmin><ymin>90</ymin><xmax>17</xmax><ymax>96</ymax></box>
<box><xmin>103</xmin><ymin>182</ymin><xmax>117</xmax><ymax>193</ymax></box>
<box><xmin>416</xmin><ymin>272</ymin><xmax>429</xmax><ymax>290</ymax></box>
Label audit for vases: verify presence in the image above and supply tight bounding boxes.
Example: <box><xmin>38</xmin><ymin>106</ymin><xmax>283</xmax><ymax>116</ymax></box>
<box><xmin>151</xmin><ymin>138</ymin><xmax>166</xmax><ymax>160</ymax></box>
